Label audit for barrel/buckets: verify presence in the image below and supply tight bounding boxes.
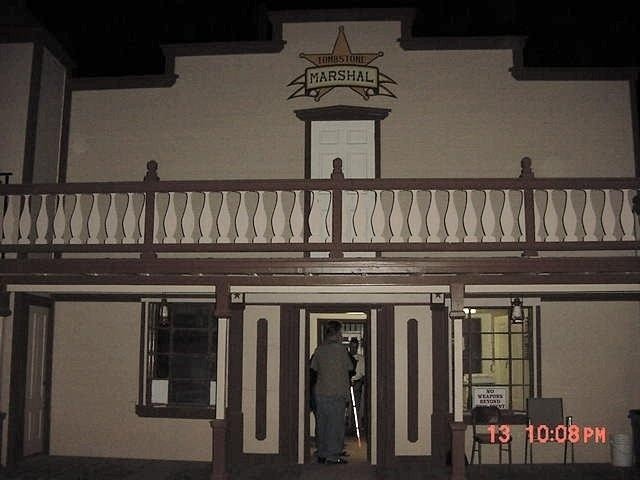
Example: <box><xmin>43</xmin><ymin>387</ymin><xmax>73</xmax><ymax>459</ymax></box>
<box><xmin>609</xmin><ymin>432</ymin><xmax>634</xmax><ymax>467</ymax></box>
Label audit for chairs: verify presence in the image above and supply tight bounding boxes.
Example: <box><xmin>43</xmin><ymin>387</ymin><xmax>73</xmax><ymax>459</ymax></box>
<box><xmin>525</xmin><ymin>398</ymin><xmax>574</xmax><ymax>467</ymax></box>
<box><xmin>469</xmin><ymin>405</ymin><xmax>512</xmax><ymax>469</ymax></box>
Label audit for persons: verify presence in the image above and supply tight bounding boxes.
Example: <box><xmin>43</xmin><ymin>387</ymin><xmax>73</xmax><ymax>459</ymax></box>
<box><xmin>309</xmin><ymin>320</ymin><xmax>366</xmax><ymax>465</ymax></box>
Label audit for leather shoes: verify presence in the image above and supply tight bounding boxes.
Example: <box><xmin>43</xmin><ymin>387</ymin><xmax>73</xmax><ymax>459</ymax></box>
<box><xmin>317</xmin><ymin>456</ymin><xmax>349</xmax><ymax>464</ymax></box>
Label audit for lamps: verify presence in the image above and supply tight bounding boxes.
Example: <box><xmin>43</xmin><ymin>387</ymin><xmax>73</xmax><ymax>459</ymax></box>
<box><xmin>158</xmin><ymin>298</ymin><xmax>171</xmax><ymax>326</ymax></box>
<box><xmin>507</xmin><ymin>295</ymin><xmax>526</xmax><ymax>324</ymax></box>
